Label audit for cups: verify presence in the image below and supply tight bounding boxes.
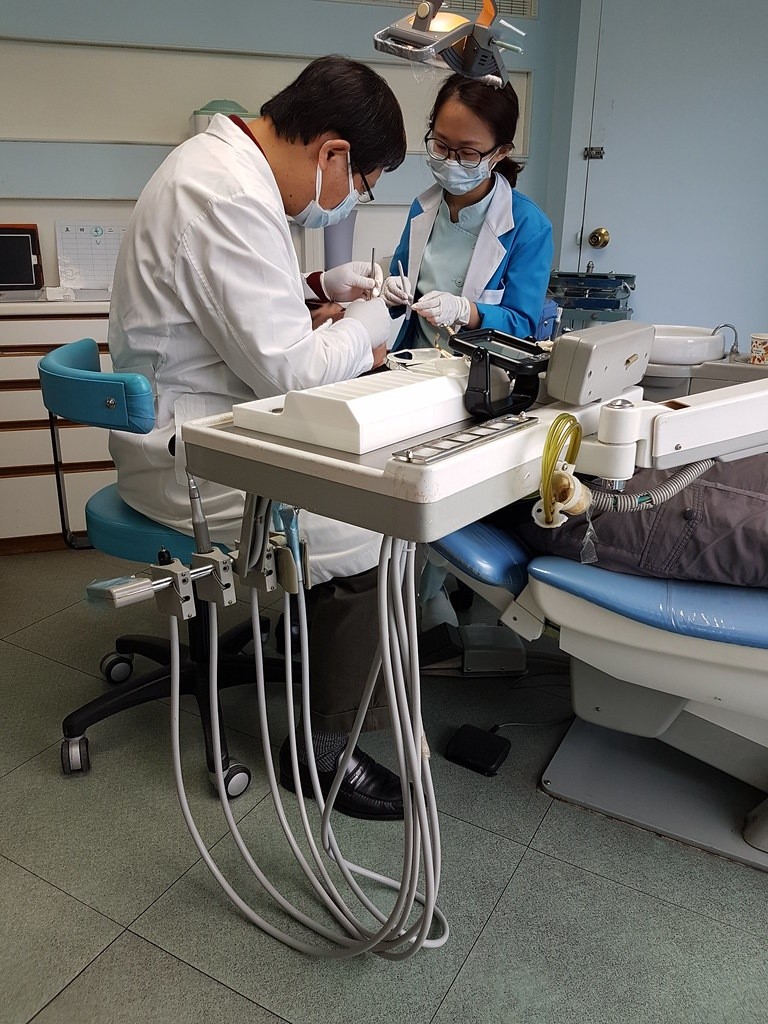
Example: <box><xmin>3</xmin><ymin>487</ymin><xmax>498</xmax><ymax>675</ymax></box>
<box><xmin>750</xmin><ymin>333</ymin><xmax>768</xmax><ymax>364</ymax></box>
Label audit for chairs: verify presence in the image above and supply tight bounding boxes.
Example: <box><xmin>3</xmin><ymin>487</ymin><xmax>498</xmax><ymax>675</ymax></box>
<box><xmin>38</xmin><ymin>338</ymin><xmax>304</xmax><ymax>800</ymax></box>
<box><xmin>427</xmin><ymin>524</ymin><xmax>767</xmax><ymax>871</ymax></box>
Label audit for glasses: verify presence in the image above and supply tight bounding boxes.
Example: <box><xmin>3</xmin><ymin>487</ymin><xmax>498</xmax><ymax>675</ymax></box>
<box><xmin>351</xmin><ymin>157</ymin><xmax>375</xmax><ymax>203</ymax></box>
<box><xmin>425</xmin><ymin>128</ymin><xmax>502</xmax><ymax>169</ymax></box>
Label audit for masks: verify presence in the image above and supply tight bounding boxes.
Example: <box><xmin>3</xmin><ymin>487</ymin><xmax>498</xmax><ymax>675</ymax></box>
<box><xmin>422</xmin><ymin>145</ymin><xmax>504</xmax><ymax>195</ymax></box>
<box><xmin>291</xmin><ymin>150</ymin><xmax>361</xmax><ymax>229</ymax></box>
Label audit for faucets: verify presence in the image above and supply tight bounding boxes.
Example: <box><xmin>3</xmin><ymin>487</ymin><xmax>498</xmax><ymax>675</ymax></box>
<box><xmin>710</xmin><ymin>322</ymin><xmax>739</xmax><ymax>355</ymax></box>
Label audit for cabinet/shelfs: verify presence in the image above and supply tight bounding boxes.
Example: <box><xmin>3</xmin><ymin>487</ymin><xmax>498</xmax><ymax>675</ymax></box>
<box><xmin>636</xmin><ymin>364</ymin><xmax>768</xmax><ymax>403</ymax></box>
<box><xmin>0</xmin><ymin>300</ymin><xmax>120</xmax><ymax>556</ymax></box>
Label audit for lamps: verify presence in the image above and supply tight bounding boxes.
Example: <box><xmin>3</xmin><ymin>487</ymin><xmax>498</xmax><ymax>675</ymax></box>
<box><xmin>373</xmin><ymin>0</ymin><xmax>523</xmax><ymax>89</ymax></box>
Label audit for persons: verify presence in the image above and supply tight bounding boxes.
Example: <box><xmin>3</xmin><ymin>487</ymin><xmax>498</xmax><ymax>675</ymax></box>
<box><xmin>381</xmin><ymin>74</ymin><xmax>559</xmax><ymax>629</ymax></box>
<box><xmin>106</xmin><ymin>52</ymin><xmax>419</xmax><ymax>825</ymax></box>
<box><xmin>303</xmin><ymin>292</ymin><xmax>768</xmax><ymax>585</ymax></box>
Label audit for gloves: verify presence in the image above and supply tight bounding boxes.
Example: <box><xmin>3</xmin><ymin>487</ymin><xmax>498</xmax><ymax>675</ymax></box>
<box><xmin>410</xmin><ymin>289</ymin><xmax>471</xmax><ymax>329</ymax></box>
<box><xmin>379</xmin><ymin>275</ymin><xmax>413</xmax><ymax>306</ymax></box>
<box><xmin>344</xmin><ymin>298</ymin><xmax>391</xmax><ymax>348</ymax></box>
<box><xmin>319</xmin><ymin>261</ymin><xmax>383</xmax><ymax>302</ymax></box>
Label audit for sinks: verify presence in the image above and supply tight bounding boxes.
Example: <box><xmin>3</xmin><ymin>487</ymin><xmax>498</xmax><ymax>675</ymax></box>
<box><xmin>649</xmin><ymin>325</ymin><xmax>725</xmax><ymax>365</ymax></box>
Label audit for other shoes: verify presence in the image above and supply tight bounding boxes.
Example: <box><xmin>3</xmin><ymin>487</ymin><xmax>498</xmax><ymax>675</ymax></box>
<box><xmin>421</xmin><ymin>584</ymin><xmax>460</xmax><ymax>634</ymax></box>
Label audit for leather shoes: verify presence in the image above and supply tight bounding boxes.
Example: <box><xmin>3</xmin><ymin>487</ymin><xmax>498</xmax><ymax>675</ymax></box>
<box><xmin>278</xmin><ymin>736</ymin><xmax>426</xmax><ymax>820</ymax></box>
<box><xmin>274</xmin><ymin>614</ymin><xmax>303</xmax><ymax>655</ymax></box>
